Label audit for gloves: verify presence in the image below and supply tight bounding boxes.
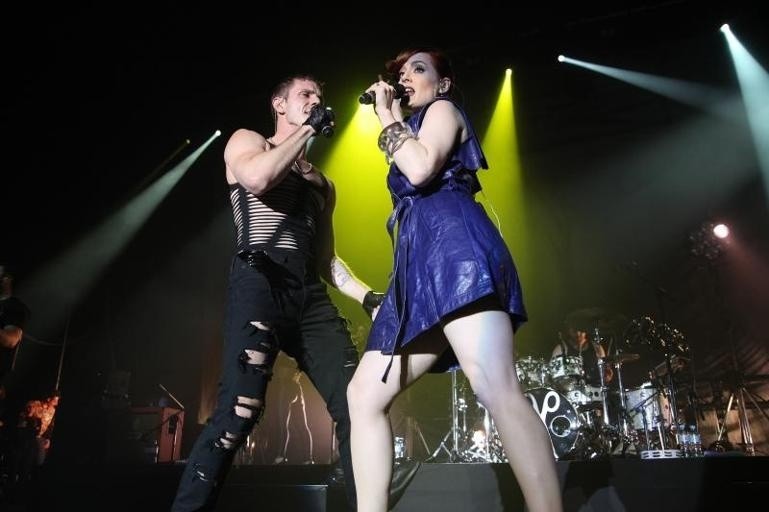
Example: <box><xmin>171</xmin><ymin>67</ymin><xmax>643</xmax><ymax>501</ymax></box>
<box><xmin>301</xmin><ymin>103</ymin><xmax>337</xmax><ymax>137</ymax></box>
<box><xmin>361</xmin><ymin>288</ymin><xmax>386</xmax><ymax>320</ymax></box>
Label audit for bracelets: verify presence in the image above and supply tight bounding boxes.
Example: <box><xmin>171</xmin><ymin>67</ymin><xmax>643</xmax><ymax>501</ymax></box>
<box><xmin>378</xmin><ymin>121</ymin><xmax>413</xmax><ymax>157</ymax></box>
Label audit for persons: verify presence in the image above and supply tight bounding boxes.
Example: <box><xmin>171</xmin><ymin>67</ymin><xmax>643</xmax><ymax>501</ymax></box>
<box><xmin>270</xmin><ymin>353</ymin><xmax>315</xmax><ymax>467</ymax></box>
<box><xmin>549</xmin><ymin>309</ymin><xmax>616</xmax><ymax>384</ymax></box>
<box><xmin>19</xmin><ymin>388</ymin><xmax>62</xmax><ymax>453</ymax></box>
<box><xmin>346</xmin><ymin>44</ymin><xmax>563</xmax><ymax>510</ymax></box>
<box><xmin>0</xmin><ymin>260</ymin><xmax>30</xmax><ymax>406</ymax></box>
<box><xmin>172</xmin><ymin>69</ymin><xmax>387</xmax><ymax>511</ymax></box>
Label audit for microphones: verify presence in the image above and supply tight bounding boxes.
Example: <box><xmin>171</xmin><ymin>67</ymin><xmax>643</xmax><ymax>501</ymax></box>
<box><xmin>158</xmin><ymin>384</ymin><xmax>170</xmax><ymax>395</ymax></box>
<box><xmin>311</xmin><ymin>106</ymin><xmax>333</xmax><ymax>138</ymax></box>
<box><xmin>594</xmin><ymin>328</ymin><xmax>600</xmax><ymax>344</ymax></box>
<box><xmin>359</xmin><ymin>84</ymin><xmax>404</xmax><ymax>104</ymax></box>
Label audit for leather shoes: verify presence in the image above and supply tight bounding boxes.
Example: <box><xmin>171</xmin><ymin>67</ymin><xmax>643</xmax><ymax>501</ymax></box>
<box><xmin>390</xmin><ymin>458</ymin><xmax>422</xmax><ymax>498</ymax></box>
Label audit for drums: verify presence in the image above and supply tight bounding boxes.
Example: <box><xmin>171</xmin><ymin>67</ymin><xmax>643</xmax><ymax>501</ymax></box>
<box><xmin>617</xmin><ymin>386</ymin><xmax>661</xmax><ymax>433</ymax></box>
<box><xmin>523</xmin><ymin>388</ymin><xmax>580</xmax><ymax>462</ymax></box>
<box><xmin>568</xmin><ymin>384</ymin><xmax>605</xmax><ymax>408</ymax></box>
<box><xmin>550</xmin><ymin>354</ymin><xmax>584</xmax><ymax>391</ymax></box>
<box><xmin>514</xmin><ymin>354</ymin><xmax>549</xmax><ymax>387</ymax></box>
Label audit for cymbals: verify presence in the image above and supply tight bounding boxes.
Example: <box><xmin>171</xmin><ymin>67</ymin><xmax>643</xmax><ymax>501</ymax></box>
<box><xmin>567</xmin><ymin>308</ymin><xmax>629</xmax><ymax>336</ymax></box>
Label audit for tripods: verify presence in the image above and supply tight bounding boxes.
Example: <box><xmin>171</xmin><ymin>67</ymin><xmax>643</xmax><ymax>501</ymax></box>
<box><xmin>583</xmin><ymin>366</ymin><xmax>668</xmax><ymax>458</ymax></box>
<box><xmin>424</xmin><ymin>369</ymin><xmax>485</xmax><ymax>462</ymax></box>
<box><xmin>715</xmin><ymin>386</ymin><xmax>769</xmax><ymax>452</ymax></box>
